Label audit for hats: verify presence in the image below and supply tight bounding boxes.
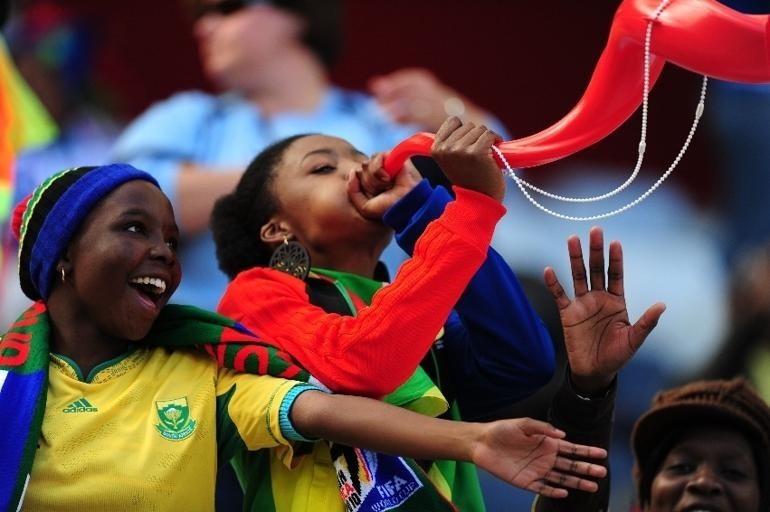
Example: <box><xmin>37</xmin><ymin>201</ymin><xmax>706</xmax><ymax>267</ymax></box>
<box><xmin>629</xmin><ymin>374</ymin><xmax>770</xmax><ymax>512</ymax></box>
<box><xmin>10</xmin><ymin>161</ymin><xmax>162</xmax><ymax>302</ymax></box>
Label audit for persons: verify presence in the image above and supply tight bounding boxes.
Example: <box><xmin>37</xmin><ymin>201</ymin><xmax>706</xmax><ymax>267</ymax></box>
<box><xmin>1</xmin><ymin>1</ymin><xmax>770</xmax><ymax>511</ymax></box>
